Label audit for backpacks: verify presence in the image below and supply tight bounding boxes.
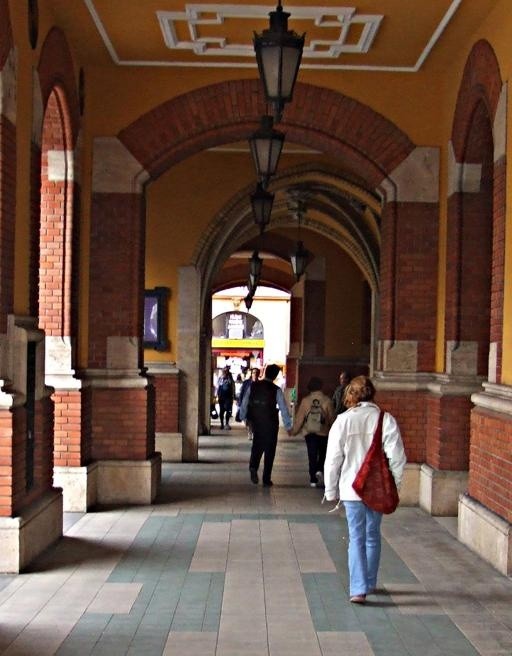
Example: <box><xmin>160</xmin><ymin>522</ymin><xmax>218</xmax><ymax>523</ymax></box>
<box><xmin>305</xmin><ymin>392</ymin><xmax>328</xmax><ymax>432</ymax></box>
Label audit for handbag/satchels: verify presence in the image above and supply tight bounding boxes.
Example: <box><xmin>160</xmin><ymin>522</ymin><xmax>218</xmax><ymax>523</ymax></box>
<box><xmin>352</xmin><ymin>434</ymin><xmax>399</xmax><ymax>515</ymax></box>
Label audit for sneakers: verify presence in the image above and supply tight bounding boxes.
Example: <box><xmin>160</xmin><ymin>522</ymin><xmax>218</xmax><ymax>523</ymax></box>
<box><xmin>316</xmin><ymin>471</ymin><xmax>323</xmax><ymax>483</ymax></box>
<box><xmin>310</xmin><ymin>483</ymin><xmax>316</xmax><ymax>487</ymax></box>
<box><xmin>264</xmin><ymin>480</ymin><xmax>273</xmax><ymax>487</ymax></box>
<box><xmin>349</xmin><ymin>594</ymin><xmax>367</xmax><ymax>603</ymax></box>
<box><xmin>250</xmin><ymin>471</ymin><xmax>258</xmax><ymax>484</ymax></box>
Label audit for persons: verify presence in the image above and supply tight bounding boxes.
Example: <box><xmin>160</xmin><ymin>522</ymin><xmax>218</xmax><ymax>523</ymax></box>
<box><xmin>322</xmin><ymin>375</ymin><xmax>408</xmax><ymax>604</ymax></box>
<box><xmin>332</xmin><ymin>371</ymin><xmax>353</xmax><ymax>413</ymax></box>
<box><xmin>216</xmin><ymin>368</ymin><xmax>260</xmax><ymax>440</ymax></box>
<box><xmin>291</xmin><ymin>376</ymin><xmax>337</xmax><ymax>488</ymax></box>
<box><xmin>238</xmin><ymin>364</ymin><xmax>292</xmax><ymax>487</ymax></box>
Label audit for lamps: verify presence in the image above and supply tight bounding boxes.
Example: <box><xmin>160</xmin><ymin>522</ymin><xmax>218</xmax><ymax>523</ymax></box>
<box><xmin>248</xmin><ymin>101</ymin><xmax>285</xmax><ymax>189</ymax></box>
<box><xmin>248</xmin><ymin>179</ymin><xmax>276</xmax><ymax>239</ymax></box>
<box><xmin>244</xmin><ymin>246</ymin><xmax>264</xmax><ymax>312</ymax></box>
<box><xmin>286</xmin><ymin>198</ymin><xmax>316</xmax><ymax>283</ymax></box>
<box><xmin>251</xmin><ymin>0</ymin><xmax>306</xmax><ymax>124</ymax></box>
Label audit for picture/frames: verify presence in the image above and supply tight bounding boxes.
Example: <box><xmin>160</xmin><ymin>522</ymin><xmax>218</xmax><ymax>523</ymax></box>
<box><xmin>144</xmin><ymin>285</ymin><xmax>171</xmax><ymax>351</ymax></box>
